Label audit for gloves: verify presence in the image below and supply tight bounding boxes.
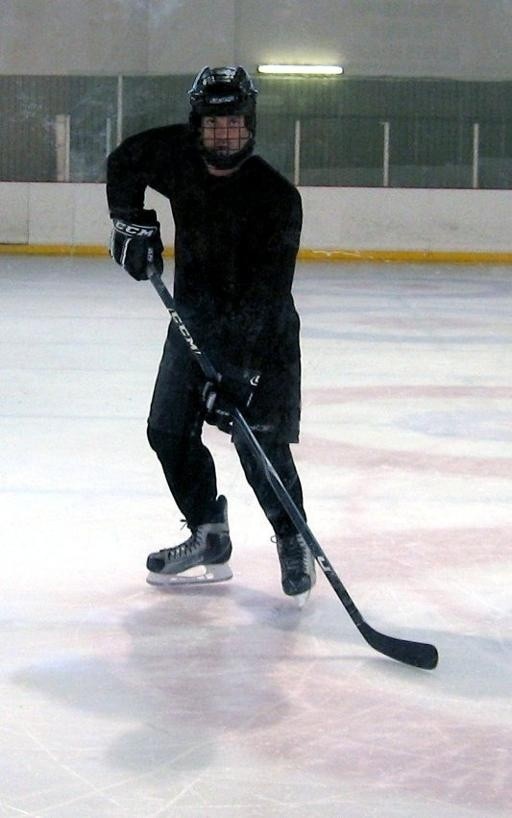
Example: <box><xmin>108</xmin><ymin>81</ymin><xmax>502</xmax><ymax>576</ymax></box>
<box><xmin>197</xmin><ymin>360</ymin><xmax>262</xmax><ymax>436</ymax></box>
<box><xmin>107</xmin><ymin>206</ymin><xmax>165</xmax><ymax>281</ymax></box>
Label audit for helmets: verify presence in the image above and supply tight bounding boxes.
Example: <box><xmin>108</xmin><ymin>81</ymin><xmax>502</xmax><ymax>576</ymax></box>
<box><xmin>186</xmin><ymin>64</ymin><xmax>260</xmax><ymax>169</ymax></box>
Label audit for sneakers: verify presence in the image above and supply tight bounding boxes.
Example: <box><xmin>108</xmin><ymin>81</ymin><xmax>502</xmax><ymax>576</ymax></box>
<box><xmin>146</xmin><ymin>495</ymin><xmax>233</xmax><ymax>574</ymax></box>
<box><xmin>275</xmin><ymin>531</ymin><xmax>317</xmax><ymax>596</ymax></box>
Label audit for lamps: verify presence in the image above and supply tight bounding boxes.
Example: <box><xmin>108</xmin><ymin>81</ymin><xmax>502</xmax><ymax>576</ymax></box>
<box><xmin>255</xmin><ymin>64</ymin><xmax>345</xmax><ymax>76</ymax></box>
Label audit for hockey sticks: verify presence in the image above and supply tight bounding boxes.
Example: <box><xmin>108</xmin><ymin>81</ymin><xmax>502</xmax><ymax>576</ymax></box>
<box><xmin>147</xmin><ymin>264</ymin><xmax>439</xmax><ymax>669</ymax></box>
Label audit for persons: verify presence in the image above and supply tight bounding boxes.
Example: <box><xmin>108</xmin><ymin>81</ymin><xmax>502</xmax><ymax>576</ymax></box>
<box><xmin>108</xmin><ymin>63</ymin><xmax>315</xmax><ymax>596</ymax></box>
